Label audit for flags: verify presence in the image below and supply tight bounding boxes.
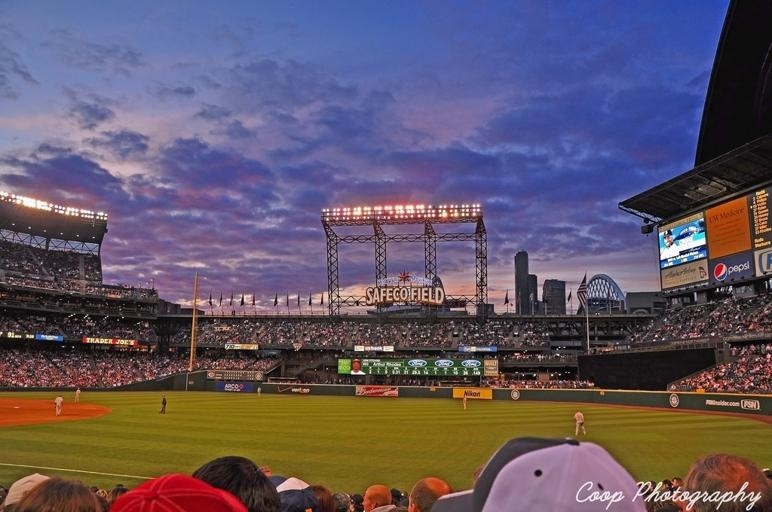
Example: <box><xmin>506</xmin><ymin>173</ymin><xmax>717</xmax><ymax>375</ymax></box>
<box><xmin>209</xmin><ymin>291</ymin><xmax>325</xmax><ymax>305</ymax></box>
<box><xmin>503</xmin><ymin>288</ymin><xmax>572</xmax><ymax>305</ymax></box>
<box><xmin>606</xmin><ymin>286</ymin><xmax>610</xmax><ymax>304</ymax></box>
<box><xmin>577</xmin><ymin>272</ymin><xmax>587</xmax><ymax>309</ymax></box>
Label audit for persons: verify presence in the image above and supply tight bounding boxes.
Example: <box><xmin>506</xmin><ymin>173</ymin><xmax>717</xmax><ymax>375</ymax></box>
<box><xmin>257</xmin><ymin>385</ymin><xmax>261</xmax><ymax>396</ymax></box>
<box><xmin>55</xmin><ymin>394</ymin><xmax>65</xmax><ymax>416</ymax></box>
<box><xmin>632</xmin><ymin>293</ymin><xmax>771</xmax><ymax>394</ymax></box>
<box><xmin>573</xmin><ymin>409</ymin><xmax>586</xmax><ymax>436</ymax></box>
<box><xmin>462</xmin><ymin>393</ymin><xmax>466</xmax><ymax>410</ymax></box>
<box><xmin>660</xmin><ymin>229</ymin><xmax>679</xmax><ymax>260</ymax></box>
<box><xmin>75</xmin><ymin>387</ymin><xmax>81</xmax><ymax>403</ymax></box>
<box><xmin>160</xmin><ymin>394</ymin><xmax>167</xmax><ymax>414</ymax></box>
<box><xmin>1</xmin><ymin>237</ymin><xmax>178</xmax><ymax>388</ymax></box>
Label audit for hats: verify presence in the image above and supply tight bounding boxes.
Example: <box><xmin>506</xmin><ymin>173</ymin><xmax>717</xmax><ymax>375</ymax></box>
<box><xmin>1</xmin><ymin>472</ymin><xmax>51</xmax><ymax>512</ymax></box>
<box><xmin>430</xmin><ymin>437</ymin><xmax>647</xmax><ymax>511</ymax></box>
<box><xmin>108</xmin><ymin>473</ymin><xmax>248</xmax><ymax>512</ymax></box>
